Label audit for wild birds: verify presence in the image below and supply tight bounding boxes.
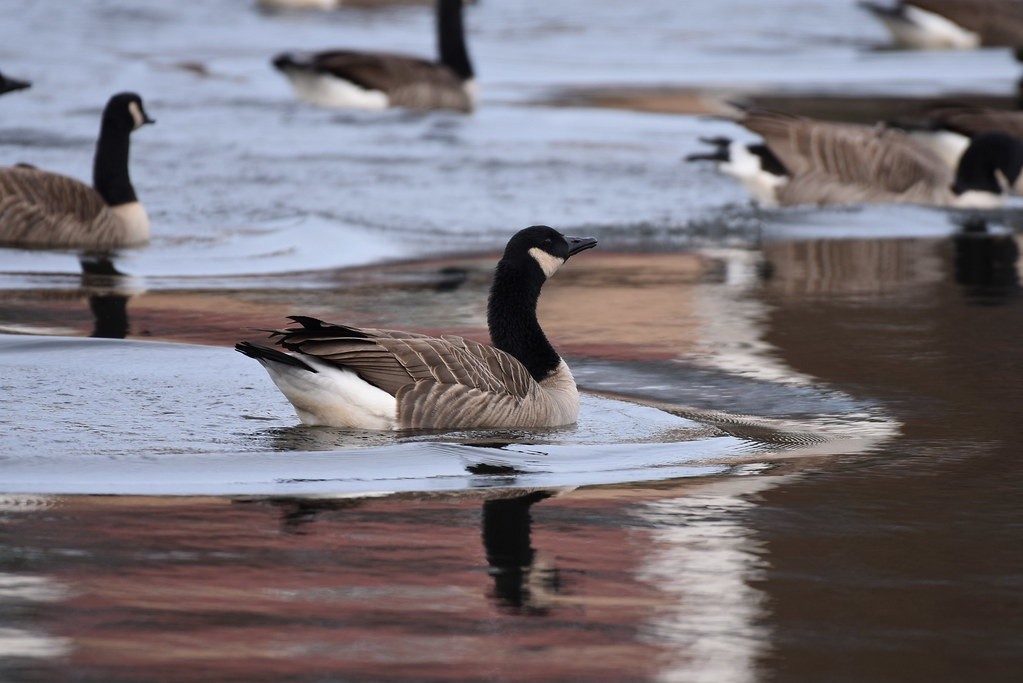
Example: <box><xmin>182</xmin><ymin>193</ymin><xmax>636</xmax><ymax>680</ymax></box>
<box><xmin>855</xmin><ymin>0</ymin><xmax>983</xmax><ymax>48</ymax></box>
<box><xmin>269</xmin><ymin>0</ymin><xmax>476</xmax><ymax>114</ymax></box>
<box><xmin>224</xmin><ymin>442</ymin><xmax>581</xmax><ymax>613</ymax></box>
<box><xmin>0</xmin><ymin>92</ymin><xmax>155</xmax><ymax>250</ymax></box>
<box><xmin>234</xmin><ymin>226</ymin><xmax>599</xmax><ymax>430</ymax></box>
<box><xmin>678</xmin><ymin>99</ymin><xmax>1023</xmax><ymax>211</ymax></box>
